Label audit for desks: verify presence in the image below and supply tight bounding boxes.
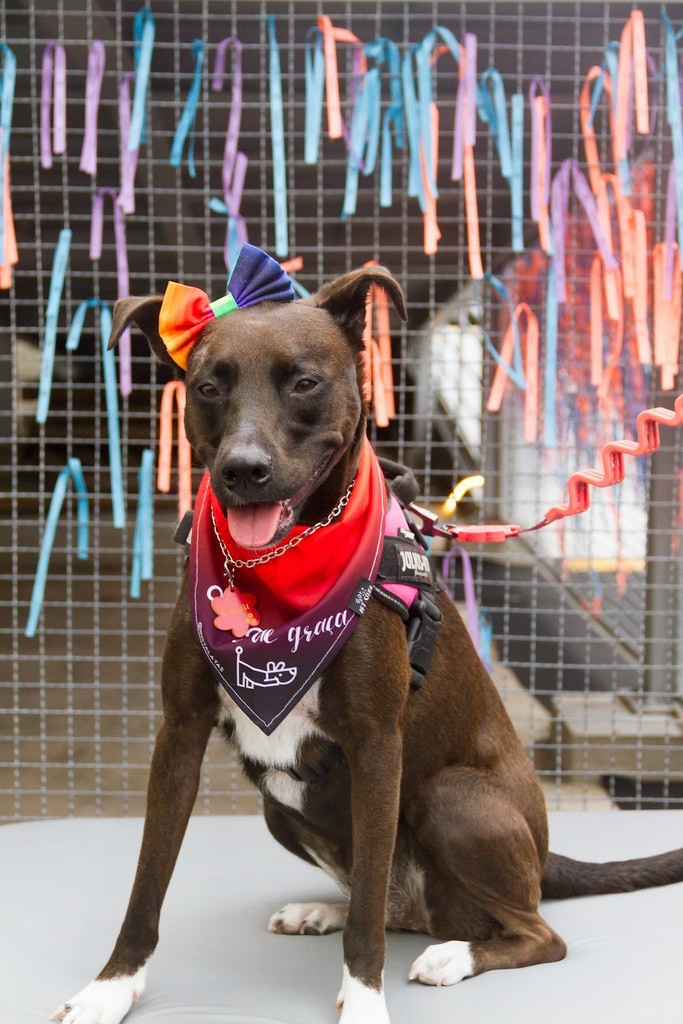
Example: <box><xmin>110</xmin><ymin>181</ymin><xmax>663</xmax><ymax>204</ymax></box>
<box><xmin>553</xmin><ymin>695</ymin><xmax>683</xmax><ymax>796</ymax></box>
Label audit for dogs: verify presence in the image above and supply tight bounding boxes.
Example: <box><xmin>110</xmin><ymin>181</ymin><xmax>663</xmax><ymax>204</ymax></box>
<box><xmin>48</xmin><ymin>242</ymin><xmax>683</xmax><ymax>1024</ymax></box>
